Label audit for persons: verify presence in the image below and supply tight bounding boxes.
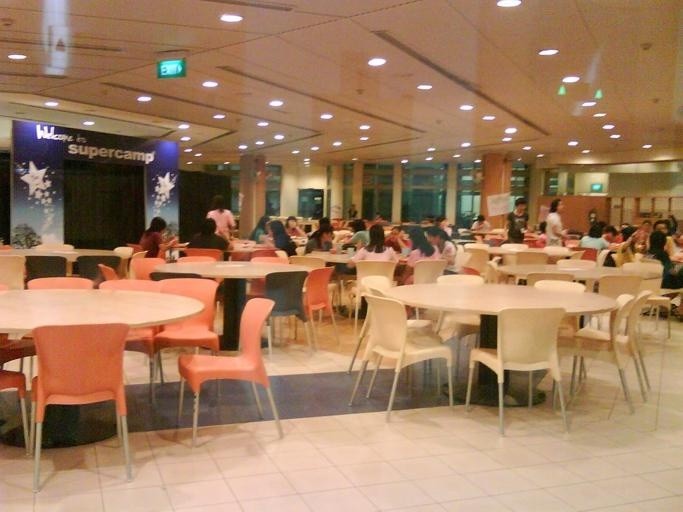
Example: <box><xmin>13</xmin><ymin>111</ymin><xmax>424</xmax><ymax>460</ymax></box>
<box><xmin>186</xmin><ymin>219</ymin><xmax>233</xmax><ymax>262</ymax></box>
<box><xmin>255</xmin><ymin>214</ymin><xmax>464</xmax><ymax>318</ymax></box>
<box><xmin>545</xmin><ymin>199</ymin><xmax>567</xmax><ymax>264</ymax></box>
<box><xmin>346</xmin><ymin>204</ymin><xmax>358</xmax><ymax>218</ymax></box>
<box><xmin>538</xmin><ymin>222</ymin><xmax>547</xmax><ymax>249</ymax></box>
<box><xmin>471</xmin><ymin>215</ymin><xmax>490</xmax><ymax>242</ymax></box>
<box><xmin>579</xmin><ymin>213</ymin><xmax>683</xmax><ymax>321</ymax></box>
<box><xmin>207</xmin><ymin>195</ymin><xmax>236</xmax><ymax>244</ymax></box>
<box><xmin>139</xmin><ymin>216</ymin><xmax>179</xmax><ymax>259</ymax></box>
<box><xmin>502</xmin><ymin>198</ymin><xmax>529</xmax><ymax>244</ymax></box>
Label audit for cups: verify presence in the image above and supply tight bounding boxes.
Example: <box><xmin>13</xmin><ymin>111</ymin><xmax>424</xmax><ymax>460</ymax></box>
<box><xmin>347</xmin><ymin>248</ymin><xmax>354</xmax><ymax>259</ymax></box>
<box><xmin>329</xmin><ymin>249</ymin><xmax>336</xmax><ymax>255</ymax></box>
<box><xmin>295</xmin><ymin>246</ymin><xmax>304</xmax><ymax>256</ymax></box>
<box><xmin>335</xmin><ymin>243</ymin><xmax>342</xmax><ymax>255</ymax></box>
<box><xmin>401</xmin><ymin>247</ymin><xmax>411</xmax><ymax>256</ymax></box>
